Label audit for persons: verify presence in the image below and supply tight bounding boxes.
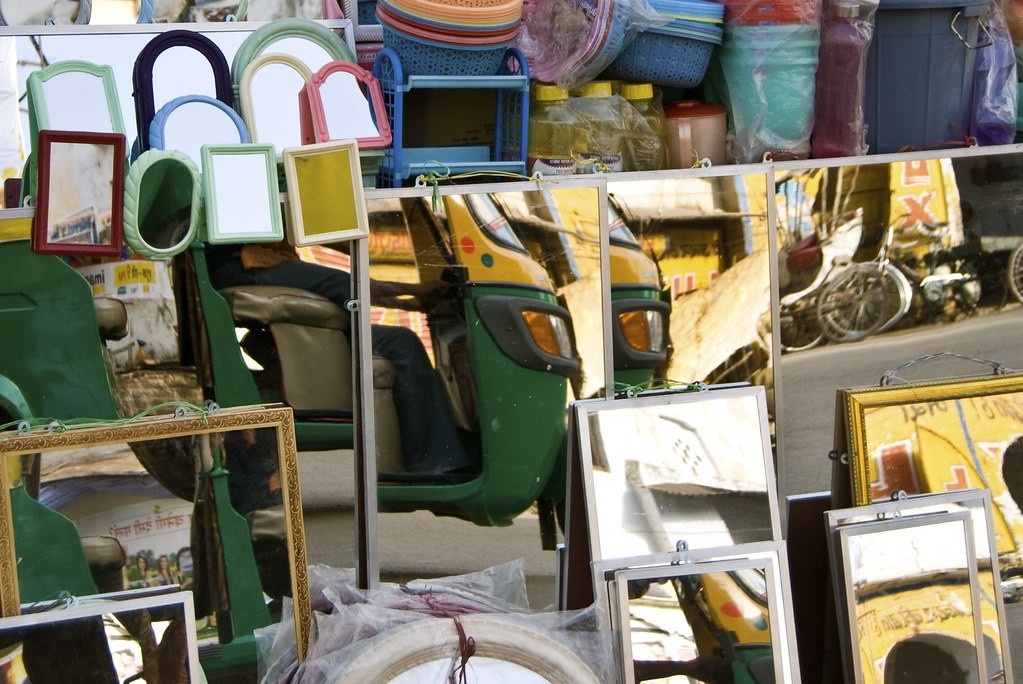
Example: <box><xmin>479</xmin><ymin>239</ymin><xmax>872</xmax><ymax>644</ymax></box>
<box><xmin>158</xmin><ymin>556</ymin><xmax>174</xmax><ymax>586</ymax></box>
<box><xmin>372</xmin><ymin>280</ymin><xmax>474</xmax><ymax>471</ymax></box>
<box><xmin>136</xmin><ymin>555</ymin><xmax>151</xmax><ymax>587</ymax></box>
<box><xmin>204</xmin><ymin>243</ymin><xmax>352</xmax><ymax>319</ymax></box>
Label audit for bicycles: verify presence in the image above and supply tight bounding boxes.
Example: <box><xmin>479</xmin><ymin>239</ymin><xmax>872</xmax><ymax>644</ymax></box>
<box><xmin>833</xmin><ymin>212</ymin><xmax>1007</xmax><ymax>334</ymax></box>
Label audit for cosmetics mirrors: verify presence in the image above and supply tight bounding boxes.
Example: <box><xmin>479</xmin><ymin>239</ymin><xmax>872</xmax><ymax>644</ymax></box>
<box><xmin>146</xmin><ymin>95</ymin><xmax>251</xmax><ymax>174</ymax></box>
<box><xmin>130</xmin><ymin>136</ymin><xmax>138</xmax><ymax>165</ymax></box>
<box><xmin>27</xmin><ymin>60</ymin><xmax>130</xmax><ymax>165</ymax></box>
<box><xmin>4</xmin><ymin>153</ymin><xmax>31</xmax><ymax>207</ymax></box>
<box><xmin>134</xmin><ymin>31</ymin><xmax>234</xmax><ymax>155</ymax></box>
<box><xmin>199</xmin><ymin>143</ymin><xmax>284</xmax><ymax>243</ymax></box>
<box><xmin>281</xmin><ymin>138</ymin><xmax>369</xmax><ymax>248</ymax></box>
<box><xmin>31</xmin><ymin>129</ymin><xmax>127</xmax><ymax>257</ymax></box>
<box><xmin>125</xmin><ymin>149</ymin><xmax>202</xmax><ymax>259</ymax></box>
<box><xmin>1</xmin><ymin>0</ymin><xmax>329</xmax><ymax>26</ymax></box>
<box><xmin>230</xmin><ymin>19</ymin><xmax>358</xmax><ymax>120</ymax></box>
<box><xmin>239</xmin><ymin>55</ymin><xmax>313</xmax><ymax>162</ymax></box>
<box><xmin>304</xmin><ymin>61</ymin><xmax>393</xmax><ymax>150</ymax></box>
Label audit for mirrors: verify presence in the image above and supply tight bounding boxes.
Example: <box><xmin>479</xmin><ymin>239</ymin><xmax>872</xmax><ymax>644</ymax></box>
<box><xmin>3</xmin><ymin>19</ymin><xmax>1023</xmax><ymax>684</ymax></box>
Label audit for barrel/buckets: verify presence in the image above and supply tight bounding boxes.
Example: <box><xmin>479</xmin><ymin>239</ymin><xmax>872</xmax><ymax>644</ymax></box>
<box><xmin>867</xmin><ymin>0</ymin><xmax>991</xmax><ymax>155</ymax></box>
<box><xmin>720</xmin><ymin>1</ymin><xmax>824</xmax><ymax>164</ymax></box>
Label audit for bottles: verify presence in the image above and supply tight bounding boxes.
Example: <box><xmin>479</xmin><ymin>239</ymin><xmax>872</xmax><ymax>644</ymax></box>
<box><xmin>526</xmin><ymin>77</ymin><xmax>666</xmax><ymax>179</ymax></box>
<box><xmin>811</xmin><ymin>0</ymin><xmax>870</xmax><ymax>160</ymax></box>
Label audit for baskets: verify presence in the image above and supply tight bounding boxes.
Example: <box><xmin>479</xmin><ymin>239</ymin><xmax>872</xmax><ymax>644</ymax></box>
<box><xmin>374</xmin><ymin>0</ymin><xmax>727</xmax><ymax>89</ymax></box>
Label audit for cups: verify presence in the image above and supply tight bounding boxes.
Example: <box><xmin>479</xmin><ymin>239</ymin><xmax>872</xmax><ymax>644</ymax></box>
<box><xmin>666</xmin><ymin>100</ymin><xmax>729</xmax><ymax>169</ymax></box>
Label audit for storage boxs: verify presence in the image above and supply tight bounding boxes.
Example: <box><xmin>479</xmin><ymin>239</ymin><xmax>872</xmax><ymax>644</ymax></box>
<box><xmin>375</xmin><ymin>1</ymin><xmax>1018</xmax><ymax>156</ymax></box>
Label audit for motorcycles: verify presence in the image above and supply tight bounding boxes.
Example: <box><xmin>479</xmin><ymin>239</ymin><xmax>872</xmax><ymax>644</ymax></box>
<box><xmin>0</xmin><ymin>186</ymin><xmax>775</xmax><ymax>684</ymax></box>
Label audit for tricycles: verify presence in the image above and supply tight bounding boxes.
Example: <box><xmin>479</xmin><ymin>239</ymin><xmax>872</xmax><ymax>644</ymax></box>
<box><xmin>779</xmin><ymin>168</ymin><xmax>863</xmax><ymax>354</ymax></box>
